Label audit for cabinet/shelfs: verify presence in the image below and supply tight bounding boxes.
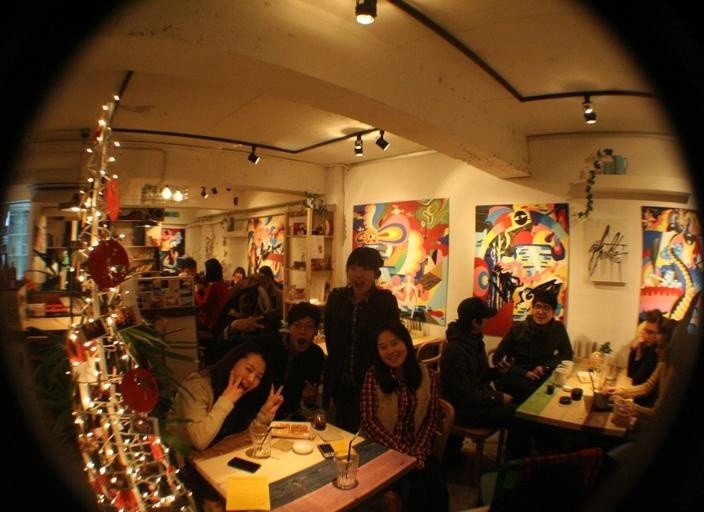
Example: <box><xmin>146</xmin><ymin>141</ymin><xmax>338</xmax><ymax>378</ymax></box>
<box><xmin>281</xmin><ymin>209</ymin><xmax>336</xmax><ymax>327</ymax></box>
<box><xmin>42</xmin><ymin>208</ymin><xmax>161</xmax><ymax>275</ymax></box>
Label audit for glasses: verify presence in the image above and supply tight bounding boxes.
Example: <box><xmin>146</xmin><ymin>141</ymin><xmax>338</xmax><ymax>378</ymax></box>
<box><xmin>199</xmin><ymin>187</ymin><xmax>232</xmax><ymax>200</ymax></box>
<box><xmin>246</xmin><ymin>127</ymin><xmax>389</xmax><ymax>164</ymax></box>
<box><xmin>141</xmin><ymin>183</ymin><xmax>189</xmax><ymax>206</ymax></box>
<box><xmin>354</xmin><ymin>0</ymin><xmax>381</xmax><ymax>27</ymax></box>
<box><xmin>580</xmin><ymin>94</ymin><xmax>597</xmax><ymax>125</ymax></box>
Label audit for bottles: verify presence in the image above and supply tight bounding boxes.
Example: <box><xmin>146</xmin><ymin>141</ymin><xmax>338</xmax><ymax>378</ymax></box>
<box><xmin>457</xmin><ymin>297</ymin><xmax>498</xmax><ymax>317</ymax></box>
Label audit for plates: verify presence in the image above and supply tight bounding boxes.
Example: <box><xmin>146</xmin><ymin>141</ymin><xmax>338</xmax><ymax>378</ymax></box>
<box><xmin>281</xmin><ymin>209</ymin><xmax>336</xmax><ymax>327</ymax></box>
<box><xmin>42</xmin><ymin>208</ymin><xmax>161</xmax><ymax>275</ymax></box>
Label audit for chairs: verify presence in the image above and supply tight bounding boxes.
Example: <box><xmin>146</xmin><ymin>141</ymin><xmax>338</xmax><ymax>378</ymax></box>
<box><xmin>416</xmin><ymin>348</ymin><xmax>504</xmax><ymax>487</ymax></box>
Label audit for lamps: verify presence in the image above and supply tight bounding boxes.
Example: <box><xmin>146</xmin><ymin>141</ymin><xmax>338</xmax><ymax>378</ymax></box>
<box><xmin>246</xmin><ymin>127</ymin><xmax>389</xmax><ymax>164</ymax></box>
<box><xmin>199</xmin><ymin>187</ymin><xmax>232</xmax><ymax>200</ymax></box>
<box><xmin>354</xmin><ymin>0</ymin><xmax>381</xmax><ymax>27</ymax></box>
<box><xmin>141</xmin><ymin>183</ymin><xmax>189</xmax><ymax>206</ymax></box>
<box><xmin>580</xmin><ymin>94</ymin><xmax>597</xmax><ymax>125</ymax></box>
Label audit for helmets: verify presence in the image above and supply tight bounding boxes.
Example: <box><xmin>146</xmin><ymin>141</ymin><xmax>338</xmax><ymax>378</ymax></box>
<box><xmin>269</xmin><ymin>420</ymin><xmax>314</xmax><ymax>441</ymax></box>
<box><xmin>292</xmin><ymin>441</ymin><xmax>315</xmax><ymax>455</ymax></box>
<box><xmin>577</xmin><ymin>369</ymin><xmax>599</xmax><ymax>386</ymax></box>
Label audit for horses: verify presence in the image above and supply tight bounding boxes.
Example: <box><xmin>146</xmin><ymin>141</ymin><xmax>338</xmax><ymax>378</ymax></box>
<box><xmin>317</xmin><ymin>444</ymin><xmax>336</xmax><ymax>459</ymax></box>
<box><xmin>227</xmin><ymin>457</ymin><xmax>261</xmax><ymax>474</ymax></box>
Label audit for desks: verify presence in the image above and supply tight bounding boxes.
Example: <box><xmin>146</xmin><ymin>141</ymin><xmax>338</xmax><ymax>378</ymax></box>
<box><xmin>490</xmin><ymin>357</ymin><xmax>637</xmax><ymax>511</ymax></box>
<box><xmin>182</xmin><ymin>400</ymin><xmax>418</xmax><ymax>511</ymax></box>
<box><xmin>315</xmin><ymin>328</ymin><xmax>444</xmax><ymax>360</ymax></box>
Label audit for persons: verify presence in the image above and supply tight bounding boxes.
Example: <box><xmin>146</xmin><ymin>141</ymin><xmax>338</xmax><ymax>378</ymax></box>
<box><xmin>607</xmin><ymin>309</ymin><xmax>667</xmax><ymax>408</ymax></box>
<box><xmin>436</xmin><ymin>296</ymin><xmax>529</xmax><ymax>463</ymax></box>
<box><xmin>204</xmin><ymin>266</ymin><xmax>283</xmax><ymax>366</ymax></box>
<box><xmin>355</xmin><ymin>321</ymin><xmax>445</xmax><ymax>510</ymax></box>
<box><xmin>492</xmin><ymin>291</ymin><xmax>576</xmax><ymax>401</ymax></box>
<box><xmin>163</xmin><ymin>251</ymin><xmax>178</xmax><ymax>274</ymax></box>
<box><xmin>603</xmin><ymin>322</ymin><xmax>680</xmax><ymax>427</ymax></box>
<box><xmin>179</xmin><ymin>258</ymin><xmax>207</xmax><ymax>292</ymax></box>
<box><xmin>323</xmin><ymin>248</ymin><xmax>401</xmax><ymax>435</ymax></box>
<box><xmin>247</xmin><ymin>301</ymin><xmax>333</xmax><ymax>422</ymax></box>
<box><xmin>166</xmin><ymin>341</ymin><xmax>284</xmax><ymax>509</ymax></box>
<box><xmin>194</xmin><ymin>259</ymin><xmax>230</xmax><ymax>335</ymax></box>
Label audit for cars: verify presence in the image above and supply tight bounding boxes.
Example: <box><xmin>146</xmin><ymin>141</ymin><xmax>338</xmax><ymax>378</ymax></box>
<box><xmin>249</xmin><ymin>424</ymin><xmax>273</xmax><ymax>461</ymax></box>
<box><xmin>546</xmin><ymin>384</ymin><xmax>555</xmax><ymax>393</ymax></box>
<box><xmin>332</xmin><ymin>449</ymin><xmax>361</xmax><ymax>492</ymax></box>
<box><xmin>593</xmin><ymin>388</ymin><xmax>612</xmax><ymax>411</ymax></box>
<box><xmin>312</xmin><ymin>408</ymin><xmax>326</xmax><ymax>431</ymax></box>
<box><xmin>561</xmin><ymin>359</ymin><xmax>575</xmax><ymax>378</ymax></box>
<box><xmin>554</xmin><ymin>368</ymin><xmax>569</xmax><ymax>386</ymax></box>
<box><xmin>572</xmin><ymin>388</ymin><xmax>582</xmax><ymax>400</ymax></box>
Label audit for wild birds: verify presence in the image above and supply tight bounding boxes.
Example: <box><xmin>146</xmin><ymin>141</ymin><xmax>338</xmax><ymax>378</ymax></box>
<box><xmin>590</xmin><ymin>375</ymin><xmax>615</xmax><ymax>412</ymax></box>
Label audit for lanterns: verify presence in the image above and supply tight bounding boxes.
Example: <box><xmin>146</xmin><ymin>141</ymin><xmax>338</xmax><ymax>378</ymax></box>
<box><xmin>119</xmin><ymin>365</ymin><xmax>160</xmax><ymax>413</ymax></box>
<box><xmin>86</xmin><ymin>238</ymin><xmax>131</xmax><ymax>293</ymax></box>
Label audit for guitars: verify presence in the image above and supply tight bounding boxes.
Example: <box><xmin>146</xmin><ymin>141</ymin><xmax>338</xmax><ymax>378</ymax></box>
<box><xmin>86</xmin><ymin>238</ymin><xmax>131</xmax><ymax>293</ymax></box>
<box><xmin>119</xmin><ymin>365</ymin><xmax>160</xmax><ymax>413</ymax></box>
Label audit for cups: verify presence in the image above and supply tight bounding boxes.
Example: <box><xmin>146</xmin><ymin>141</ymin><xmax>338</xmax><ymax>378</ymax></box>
<box><xmin>534</xmin><ymin>304</ymin><xmax>553</xmax><ymax>312</ymax></box>
<box><xmin>290</xmin><ymin>322</ymin><xmax>316</xmax><ymax>327</ymax></box>
<box><xmin>645</xmin><ymin>328</ymin><xmax>656</xmax><ymax>332</ymax></box>
